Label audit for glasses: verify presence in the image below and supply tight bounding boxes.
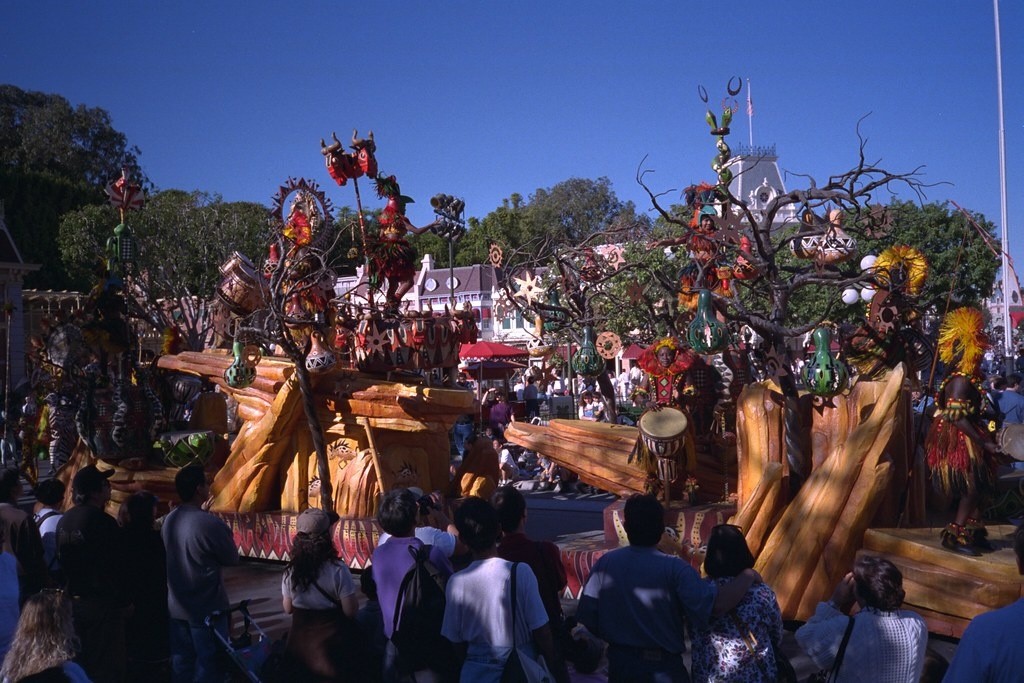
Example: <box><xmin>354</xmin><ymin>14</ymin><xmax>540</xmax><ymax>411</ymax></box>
<box><xmin>712</xmin><ymin>524</ymin><xmax>743</xmax><ymax>535</ymax></box>
<box><xmin>39</xmin><ymin>587</ymin><xmax>68</xmax><ymax>602</ymax></box>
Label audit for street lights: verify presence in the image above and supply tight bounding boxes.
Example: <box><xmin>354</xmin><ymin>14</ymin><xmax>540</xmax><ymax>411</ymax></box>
<box><xmin>429</xmin><ymin>193</ymin><xmax>466</xmax><ymax>308</ymax></box>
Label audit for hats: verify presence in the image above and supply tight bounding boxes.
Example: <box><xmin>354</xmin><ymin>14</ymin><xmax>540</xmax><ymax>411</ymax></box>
<box><xmin>298</xmin><ymin>509</ymin><xmax>340</xmax><ymax>534</ymax></box>
<box><xmin>72</xmin><ymin>464</ymin><xmax>115</xmax><ymax>489</ymax></box>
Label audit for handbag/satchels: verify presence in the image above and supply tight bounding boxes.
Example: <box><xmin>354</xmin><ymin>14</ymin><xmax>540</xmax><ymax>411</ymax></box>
<box><xmin>807</xmin><ymin>670</ymin><xmax>826</xmax><ymax>683</ymax></box>
<box><xmin>771</xmin><ymin>642</ymin><xmax>797</xmax><ymax>683</ymax></box>
<box><xmin>500</xmin><ymin>648</ymin><xmax>556</xmax><ymax>683</ymax></box>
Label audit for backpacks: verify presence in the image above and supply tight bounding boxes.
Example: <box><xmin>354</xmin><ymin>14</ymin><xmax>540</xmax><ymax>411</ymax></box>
<box><xmin>391</xmin><ymin>545</ymin><xmax>469</xmax><ymax>670</ymax></box>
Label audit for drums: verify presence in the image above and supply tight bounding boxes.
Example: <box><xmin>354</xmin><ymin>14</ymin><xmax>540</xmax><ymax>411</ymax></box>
<box><xmin>168</xmin><ymin>375</ymin><xmax>203</xmax><ymax>423</ymax></box>
<box><xmin>995</xmin><ymin>423</ymin><xmax>1024</xmax><ymax>464</ymax></box>
<box><xmin>638</xmin><ymin>406</ymin><xmax>689</xmax><ymax>487</ymax></box>
<box><xmin>215</xmin><ymin>263</ymin><xmax>270</xmax><ymax>317</ymax></box>
<box><xmin>218</xmin><ymin>250</ymin><xmax>256</xmax><ymax>278</ymax></box>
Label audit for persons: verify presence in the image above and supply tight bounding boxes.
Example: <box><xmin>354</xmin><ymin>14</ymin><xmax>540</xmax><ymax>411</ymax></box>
<box><xmin>791</xmin><ymin>357</ymin><xmax>805</xmax><ymax>384</ymax></box>
<box><xmin>981</xmin><ymin>347</ymin><xmax>1024</xmax><ymax>473</ymax></box>
<box><xmin>456</xmin><ymin>373</ymin><xmax>473</xmax><ymax>391</ymax></box>
<box><xmin>450</xmin><ymin>414</ymin><xmax>571</xmax><ymax>483</ymax></box>
<box><xmin>513</xmin><ymin>376</ymin><xmax>553</xmax><ymax>416</ymax></box>
<box><xmin>0</xmin><ymin>463</ymin><xmax>1024</xmax><ymax>683</ymax></box>
<box><xmin>647</xmin><ymin>206</ymin><xmax>733</xmax><ymax>308</ymax></box>
<box><xmin>481</xmin><ymin>386</ymin><xmax>515</xmax><ymax>430</ymax></box>
<box><xmin>0</xmin><ymin>397</ymin><xmax>40</xmax><ymax>495</ymax></box>
<box><xmin>925</xmin><ymin>335</ymin><xmax>1001</xmax><ymax>556</ymax></box>
<box><xmin>370</xmin><ymin>195</ymin><xmax>440</xmax><ymax>310</ymax></box>
<box><xmin>578</xmin><ymin>362</ymin><xmax>642</xmax><ymax>425</ymax></box>
<box><xmin>628</xmin><ymin>337</ymin><xmax>698</xmax><ymax>500</ymax></box>
<box><xmin>912</xmin><ymin>390</ymin><xmax>933</xmax><ymax>414</ymax></box>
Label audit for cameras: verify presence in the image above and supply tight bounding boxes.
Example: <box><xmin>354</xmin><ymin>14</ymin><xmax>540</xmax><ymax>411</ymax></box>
<box><xmin>416</xmin><ymin>491</ymin><xmax>443</xmax><ymax>515</ymax></box>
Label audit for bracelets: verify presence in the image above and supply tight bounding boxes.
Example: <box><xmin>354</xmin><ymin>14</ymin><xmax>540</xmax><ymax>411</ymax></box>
<box><xmin>487</xmin><ymin>390</ymin><xmax>490</xmax><ymax>394</ymax></box>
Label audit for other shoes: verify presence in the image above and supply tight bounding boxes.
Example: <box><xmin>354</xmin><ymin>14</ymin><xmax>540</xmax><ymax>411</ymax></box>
<box><xmin>964</xmin><ymin>519</ymin><xmax>998</xmax><ymax>553</ymax></box>
<box><xmin>940</xmin><ymin>523</ymin><xmax>980</xmax><ymax>555</ymax></box>
